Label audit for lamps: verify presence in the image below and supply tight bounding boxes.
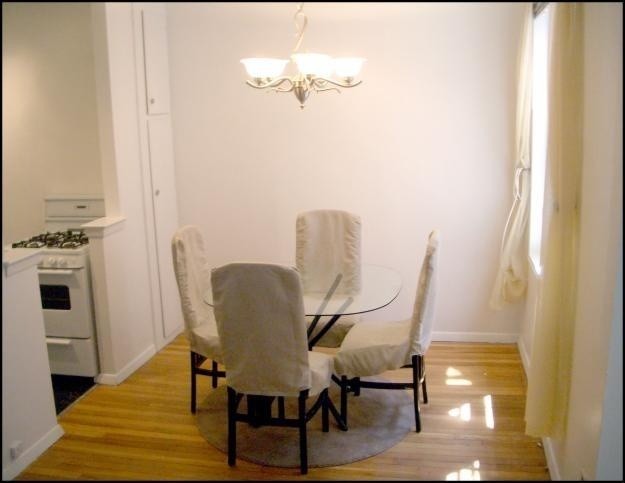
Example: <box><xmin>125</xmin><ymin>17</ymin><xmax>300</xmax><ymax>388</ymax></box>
<box><xmin>241</xmin><ymin>2</ymin><xmax>364</xmax><ymax>109</ymax></box>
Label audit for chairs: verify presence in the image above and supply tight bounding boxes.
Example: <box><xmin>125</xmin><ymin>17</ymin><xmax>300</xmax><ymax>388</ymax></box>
<box><xmin>207</xmin><ymin>262</ymin><xmax>337</xmax><ymax>477</ymax></box>
<box><xmin>294</xmin><ymin>209</ymin><xmax>363</xmax><ymax>396</ymax></box>
<box><xmin>331</xmin><ymin>227</ymin><xmax>444</xmax><ymax>433</ymax></box>
<box><xmin>169</xmin><ymin>223</ymin><xmax>223</xmax><ymax>416</ymax></box>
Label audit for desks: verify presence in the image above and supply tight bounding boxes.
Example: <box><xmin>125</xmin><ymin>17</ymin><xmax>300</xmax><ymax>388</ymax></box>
<box><xmin>199</xmin><ymin>263</ymin><xmax>405</xmax><ymax>432</ymax></box>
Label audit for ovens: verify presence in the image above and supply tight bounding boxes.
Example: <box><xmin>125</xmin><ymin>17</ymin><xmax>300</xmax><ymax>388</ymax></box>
<box><xmin>37</xmin><ymin>267</ymin><xmax>99</xmax><ymax>377</ymax></box>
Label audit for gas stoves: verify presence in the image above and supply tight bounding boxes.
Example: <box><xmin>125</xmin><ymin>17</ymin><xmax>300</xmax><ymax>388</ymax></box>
<box><xmin>11</xmin><ymin>231</ymin><xmax>90</xmax><ymax>248</ymax></box>
